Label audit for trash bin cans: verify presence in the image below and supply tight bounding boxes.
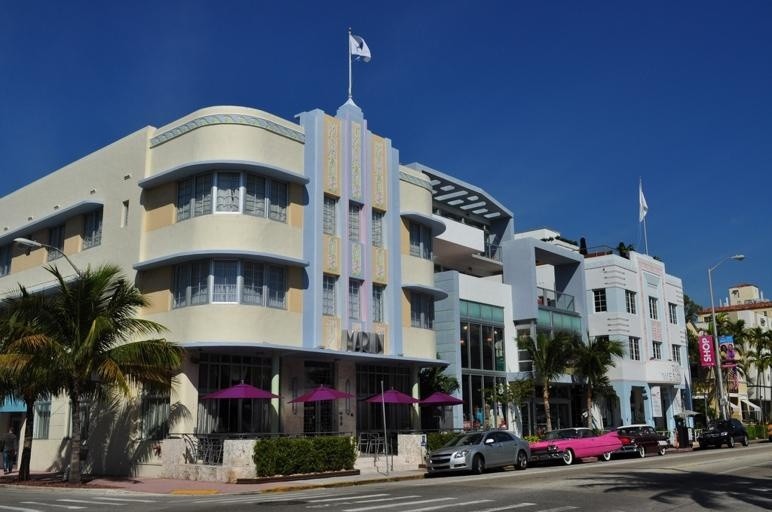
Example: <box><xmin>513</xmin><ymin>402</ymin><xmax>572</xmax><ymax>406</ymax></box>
<box><xmin>675</xmin><ymin>418</ymin><xmax>689</xmax><ymax>449</ymax></box>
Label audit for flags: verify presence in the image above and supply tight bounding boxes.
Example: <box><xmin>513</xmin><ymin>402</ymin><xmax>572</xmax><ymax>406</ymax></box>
<box><xmin>637</xmin><ymin>183</ymin><xmax>649</xmax><ymax>223</ymax></box>
<box><xmin>352</xmin><ymin>34</ymin><xmax>371</xmax><ymax>63</ymax></box>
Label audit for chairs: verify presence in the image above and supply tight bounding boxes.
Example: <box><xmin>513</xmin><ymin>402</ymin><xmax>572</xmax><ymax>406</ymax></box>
<box><xmin>358</xmin><ymin>431</ymin><xmax>393</xmax><ymax>454</ymax></box>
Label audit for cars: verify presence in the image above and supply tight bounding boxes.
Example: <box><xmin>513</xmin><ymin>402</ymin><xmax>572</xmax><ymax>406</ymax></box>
<box><xmin>610</xmin><ymin>423</ymin><xmax>670</xmax><ymax>458</ymax></box>
<box><xmin>696</xmin><ymin>418</ymin><xmax>749</xmax><ymax>448</ymax></box>
<box><xmin>528</xmin><ymin>427</ymin><xmax>630</xmax><ymax>465</ymax></box>
<box><xmin>424</xmin><ymin>428</ymin><xmax>532</xmax><ymax>474</ymax></box>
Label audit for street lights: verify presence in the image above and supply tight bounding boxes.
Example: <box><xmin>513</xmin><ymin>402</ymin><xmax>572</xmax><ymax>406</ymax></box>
<box><xmin>14</xmin><ymin>237</ymin><xmax>95</xmax><ymax>441</ymax></box>
<box><xmin>708</xmin><ymin>255</ymin><xmax>744</xmax><ymax>419</ymax></box>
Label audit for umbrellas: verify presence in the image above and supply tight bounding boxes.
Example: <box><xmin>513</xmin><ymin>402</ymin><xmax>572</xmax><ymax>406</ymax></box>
<box><xmin>363</xmin><ymin>386</ymin><xmax>419</xmax><ymax>442</ymax></box>
<box><xmin>418</xmin><ymin>390</ymin><xmax>466</xmax><ymax>434</ymax></box>
<box><xmin>288</xmin><ymin>383</ymin><xmax>354</xmax><ymax>436</ymax></box>
<box><xmin>201</xmin><ymin>380</ymin><xmax>284</xmax><ymax>439</ymax></box>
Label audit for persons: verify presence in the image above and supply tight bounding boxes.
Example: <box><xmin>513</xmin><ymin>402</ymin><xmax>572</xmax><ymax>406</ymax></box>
<box><xmin>0</xmin><ymin>426</ymin><xmax>18</xmax><ymax>474</ymax></box>
<box><xmin>721</xmin><ymin>344</ymin><xmax>736</xmax><ymax>366</ymax></box>
